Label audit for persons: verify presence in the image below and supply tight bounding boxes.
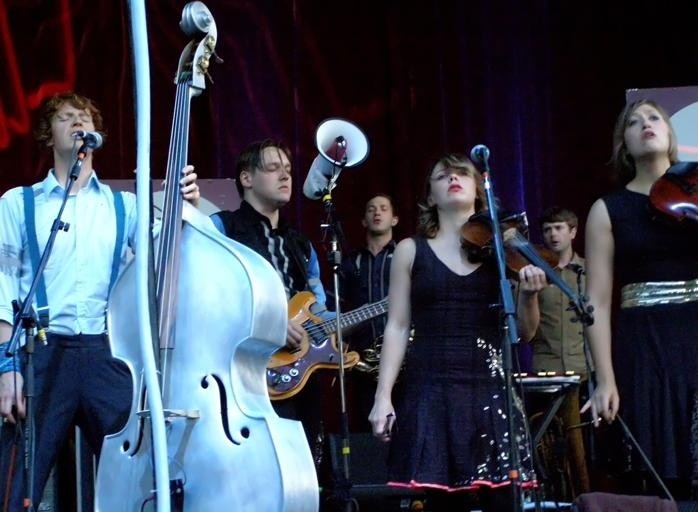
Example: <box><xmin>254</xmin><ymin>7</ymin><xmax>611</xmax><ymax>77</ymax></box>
<box><xmin>579</xmin><ymin>99</ymin><xmax>698</xmax><ymax>499</ymax></box>
<box><xmin>0</xmin><ymin>92</ymin><xmax>200</xmax><ymax>512</ymax></box>
<box><xmin>368</xmin><ymin>149</ymin><xmax>554</xmax><ymax>509</ymax></box>
<box><xmin>207</xmin><ymin>136</ymin><xmax>344</xmax><ymax>427</ymax></box>
<box><xmin>527</xmin><ymin>209</ymin><xmax>594</xmax><ymax>485</ymax></box>
<box><xmin>328</xmin><ymin>195</ymin><xmax>400</xmax><ymax>363</ymax></box>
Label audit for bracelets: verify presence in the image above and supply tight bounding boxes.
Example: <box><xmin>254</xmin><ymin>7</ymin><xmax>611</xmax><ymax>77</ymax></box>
<box><xmin>0</xmin><ymin>342</ymin><xmax>22</xmax><ymax>374</ymax></box>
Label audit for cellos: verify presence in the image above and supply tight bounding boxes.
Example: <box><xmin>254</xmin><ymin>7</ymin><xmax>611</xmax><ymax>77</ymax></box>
<box><xmin>94</xmin><ymin>1</ymin><xmax>321</xmax><ymax>512</ymax></box>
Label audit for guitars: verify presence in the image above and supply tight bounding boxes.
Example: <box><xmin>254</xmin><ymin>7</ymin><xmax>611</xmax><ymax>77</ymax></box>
<box><xmin>267</xmin><ymin>290</ymin><xmax>389</xmax><ymax>401</ymax></box>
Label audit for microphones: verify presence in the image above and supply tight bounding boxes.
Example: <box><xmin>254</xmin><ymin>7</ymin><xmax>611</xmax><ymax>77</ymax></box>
<box><xmin>468</xmin><ymin>144</ymin><xmax>492</xmax><ymax>164</ymax></box>
<box><xmin>76</xmin><ymin>131</ymin><xmax>103</xmax><ymax>149</ymax></box>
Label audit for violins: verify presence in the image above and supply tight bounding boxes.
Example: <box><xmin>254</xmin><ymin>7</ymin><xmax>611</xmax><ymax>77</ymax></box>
<box><xmin>461</xmin><ymin>210</ymin><xmax>594</xmax><ymax>326</ymax></box>
<box><xmin>644</xmin><ymin>170</ymin><xmax>698</xmax><ymax>232</ymax></box>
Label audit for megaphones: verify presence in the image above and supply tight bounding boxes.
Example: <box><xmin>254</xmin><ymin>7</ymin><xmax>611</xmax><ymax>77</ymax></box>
<box><xmin>303</xmin><ymin>118</ymin><xmax>372</xmax><ymax>201</ymax></box>
